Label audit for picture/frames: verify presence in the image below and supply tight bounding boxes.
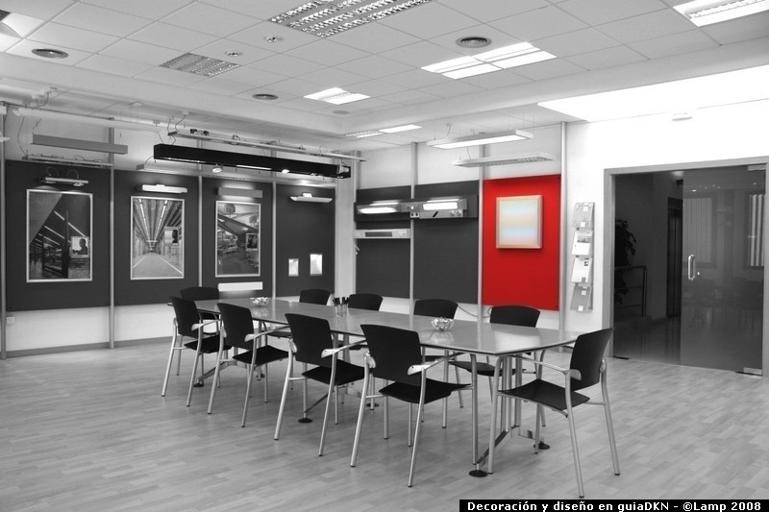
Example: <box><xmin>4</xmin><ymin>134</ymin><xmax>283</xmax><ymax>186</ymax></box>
<box><xmin>26</xmin><ymin>188</ymin><xmax>93</xmax><ymax>282</ymax></box>
<box><xmin>130</xmin><ymin>195</ymin><xmax>185</xmax><ymax>280</ymax></box>
<box><xmin>214</xmin><ymin>200</ymin><xmax>261</xmax><ymax>278</ymax></box>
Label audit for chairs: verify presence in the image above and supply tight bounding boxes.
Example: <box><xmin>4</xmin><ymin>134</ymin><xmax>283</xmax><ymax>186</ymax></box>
<box><xmin>451</xmin><ymin>305</ymin><xmax>549</xmax><ymax>430</ymax></box>
<box><xmin>348</xmin><ymin>293</ymin><xmax>382</xmax><ymax>311</ymax></box>
<box><xmin>396</xmin><ymin>298</ymin><xmax>465</xmax><ymax>427</ymax></box>
<box><xmin>350</xmin><ymin>324</ymin><xmax>477</xmax><ymax>487</ymax></box>
<box><xmin>496</xmin><ymin>326</ymin><xmax>621</xmax><ymax>500</ymax></box>
<box><xmin>159</xmin><ymin>295</ymin><xmax>238</xmax><ymax>406</ymax></box>
<box><xmin>176</xmin><ymin>287</ymin><xmax>261</xmax><ymax>390</ymax></box>
<box><xmin>273</xmin><ymin>312</ymin><xmax>389</xmax><ymax>458</ymax></box>
<box><xmin>208</xmin><ymin>302</ymin><xmax>307</xmax><ymax>428</ymax></box>
<box><xmin>300</xmin><ymin>289</ymin><xmax>330</xmax><ymax>305</ymax></box>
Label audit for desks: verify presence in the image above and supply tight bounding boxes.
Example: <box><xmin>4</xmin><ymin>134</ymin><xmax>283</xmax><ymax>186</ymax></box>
<box><xmin>192</xmin><ymin>297</ymin><xmax>579</xmax><ymax>477</ymax></box>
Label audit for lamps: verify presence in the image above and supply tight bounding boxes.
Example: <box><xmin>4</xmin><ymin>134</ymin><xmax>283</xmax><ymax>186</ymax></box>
<box><xmin>431</xmin><ymin>129</ymin><xmax>535</xmax><ymax>152</ymax></box>
<box><xmin>422</xmin><ymin>197</ymin><xmax>464</xmax><ymax>211</ymax></box>
<box><xmin>43</xmin><ymin>176</ymin><xmax>89</xmax><ymax>186</ymax></box>
<box><xmin>141</xmin><ymin>183</ymin><xmax>187</xmax><ymax>195</ymax></box>
<box><xmin>355</xmin><ymin>201</ymin><xmax>399</xmax><ymax>217</ymax></box>
<box><xmin>457</xmin><ymin>151</ymin><xmax>554</xmax><ymax>169</ymax></box>
<box><xmin>212</xmin><ymin>164</ymin><xmax>224</xmax><ymax>175</ymax></box>
